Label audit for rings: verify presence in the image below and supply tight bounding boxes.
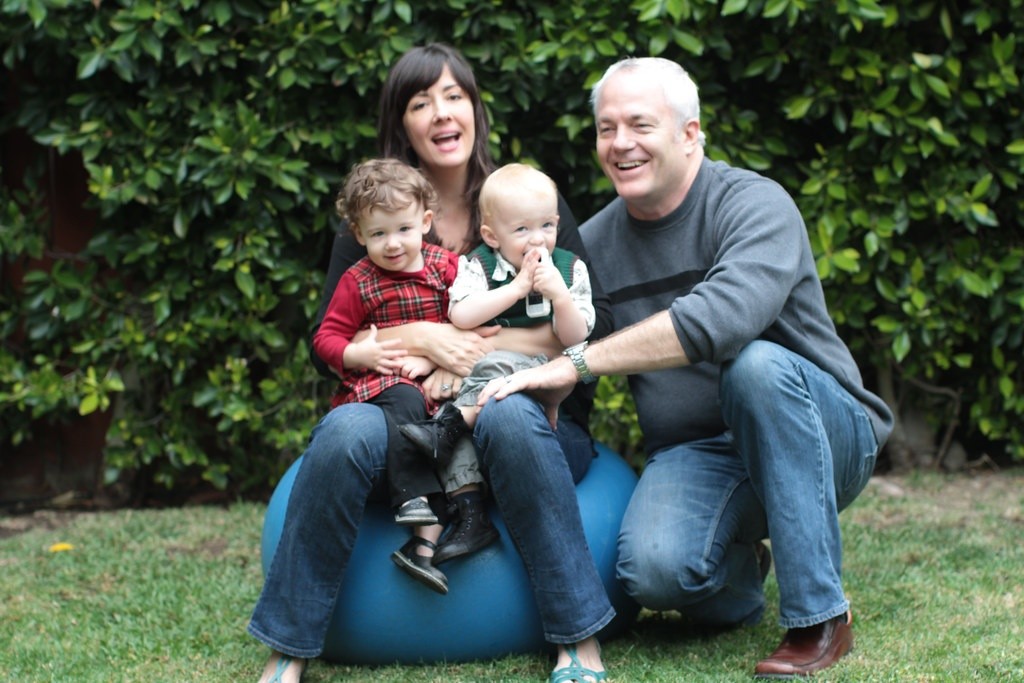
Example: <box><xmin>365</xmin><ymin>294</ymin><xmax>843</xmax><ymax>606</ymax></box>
<box><xmin>441</xmin><ymin>384</ymin><xmax>451</xmax><ymax>391</ymax></box>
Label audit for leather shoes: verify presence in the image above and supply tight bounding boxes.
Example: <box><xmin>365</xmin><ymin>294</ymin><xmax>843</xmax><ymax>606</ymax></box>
<box><xmin>394</xmin><ymin>495</ymin><xmax>438</xmax><ymax>523</ymax></box>
<box><xmin>754</xmin><ymin>612</ymin><xmax>854</xmax><ymax>680</ymax></box>
<box><xmin>392</xmin><ymin>536</ymin><xmax>448</xmax><ymax>594</ymax></box>
<box><xmin>680</xmin><ymin>542</ymin><xmax>770</xmax><ymax>642</ymax></box>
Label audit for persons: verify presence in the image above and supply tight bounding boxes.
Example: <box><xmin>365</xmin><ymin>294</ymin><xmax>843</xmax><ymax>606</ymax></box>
<box><xmin>248</xmin><ymin>44</ymin><xmax>617</xmax><ymax>683</ymax></box>
<box><xmin>314</xmin><ymin>159</ymin><xmax>460</xmax><ymax>594</ymax></box>
<box><xmin>476</xmin><ymin>58</ymin><xmax>896</xmax><ymax>680</ymax></box>
<box><xmin>397</xmin><ymin>163</ymin><xmax>597</xmax><ymax>566</ymax></box>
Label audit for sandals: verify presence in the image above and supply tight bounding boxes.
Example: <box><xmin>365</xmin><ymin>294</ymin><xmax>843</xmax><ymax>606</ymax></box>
<box><xmin>550</xmin><ymin>636</ymin><xmax>606</xmax><ymax>683</ymax></box>
<box><xmin>257</xmin><ymin>649</ymin><xmax>309</xmax><ymax>683</ymax></box>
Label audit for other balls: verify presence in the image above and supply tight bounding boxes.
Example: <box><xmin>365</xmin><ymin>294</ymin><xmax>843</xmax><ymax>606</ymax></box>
<box><xmin>258</xmin><ymin>434</ymin><xmax>642</xmax><ymax>669</ymax></box>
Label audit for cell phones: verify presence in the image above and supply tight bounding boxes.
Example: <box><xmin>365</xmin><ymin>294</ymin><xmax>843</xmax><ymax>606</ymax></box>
<box><xmin>526</xmin><ymin>289</ymin><xmax>551</xmax><ymax>319</ymax></box>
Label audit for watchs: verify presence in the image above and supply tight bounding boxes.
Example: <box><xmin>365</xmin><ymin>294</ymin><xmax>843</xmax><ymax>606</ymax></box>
<box><xmin>562</xmin><ymin>341</ymin><xmax>596</xmax><ymax>384</ymax></box>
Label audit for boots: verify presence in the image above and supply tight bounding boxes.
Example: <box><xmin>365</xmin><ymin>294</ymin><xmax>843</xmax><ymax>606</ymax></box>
<box><xmin>432</xmin><ymin>491</ymin><xmax>500</xmax><ymax>565</ymax></box>
<box><xmin>399</xmin><ymin>401</ymin><xmax>473</xmax><ymax>469</ymax></box>
